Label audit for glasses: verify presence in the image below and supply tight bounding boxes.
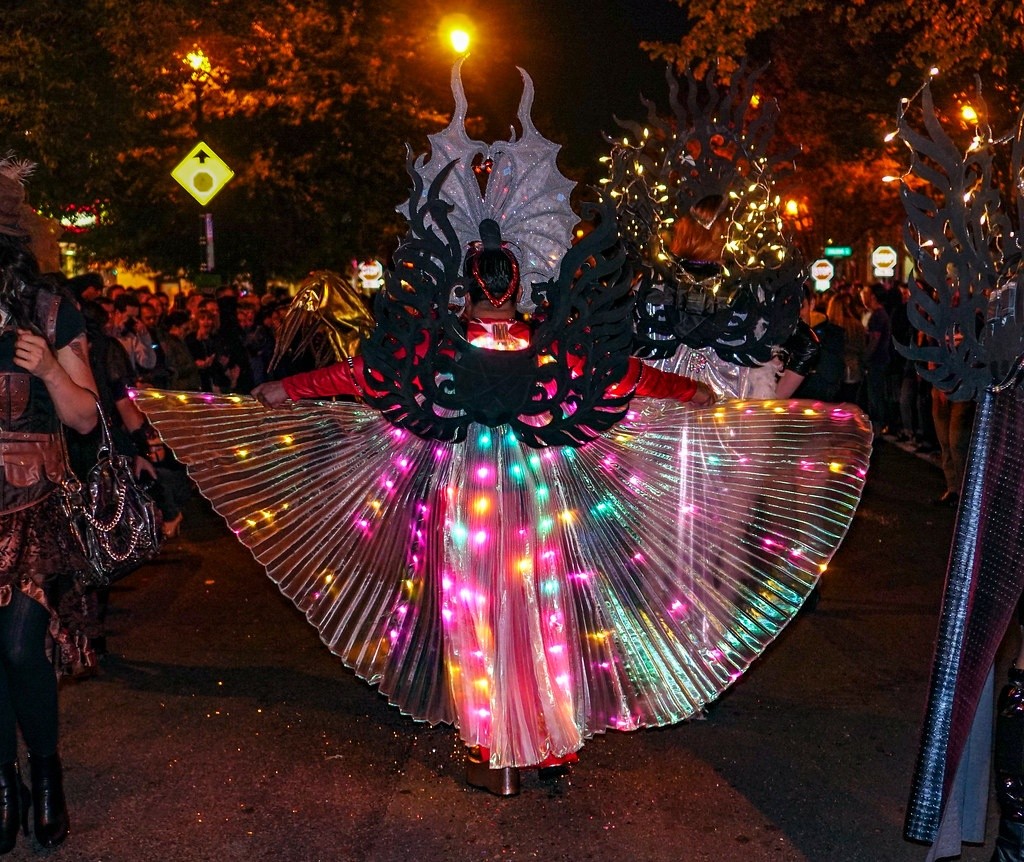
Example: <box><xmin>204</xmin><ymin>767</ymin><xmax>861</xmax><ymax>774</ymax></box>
<box><xmin>180</xmin><ymin>326</ymin><xmax>189</xmax><ymax>332</ymax></box>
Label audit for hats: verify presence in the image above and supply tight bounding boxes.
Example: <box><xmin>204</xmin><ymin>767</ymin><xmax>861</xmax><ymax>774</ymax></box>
<box><xmin>83</xmin><ymin>273</ymin><xmax>108</xmax><ymax>289</ymax></box>
<box><xmin>0</xmin><ymin>173</ymin><xmax>66</xmax><ymax>257</ymax></box>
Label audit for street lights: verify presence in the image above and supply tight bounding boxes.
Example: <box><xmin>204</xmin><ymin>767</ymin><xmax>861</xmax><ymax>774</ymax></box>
<box><xmin>182</xmin><ymin>48</ymin><xmax>211</xmax><ymax>274</ymax></box>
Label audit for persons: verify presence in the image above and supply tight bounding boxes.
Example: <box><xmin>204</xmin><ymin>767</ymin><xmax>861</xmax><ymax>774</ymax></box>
<box><xmin>252</xmin><ymin>215</ymin><xmax>716</xmax><ymax>795</ymax></box>
<box><xmin>775</xmin><ymin>256</ymin><xmax>1024</xmax><ymax>505</ymax></box>
<box><xmin>0</xmin><ymin>273</ymin><xmax>293</xmax><ymax>541</ymax></box>
<box><xmin>629</xmin><ymin>197</ymin><xmax>825</xmax><ymax>604</ymax></box>
<box><xmin>0</xmin><ymin>173</ymin><xmax>102</xmax><ymax>852</ymax></box>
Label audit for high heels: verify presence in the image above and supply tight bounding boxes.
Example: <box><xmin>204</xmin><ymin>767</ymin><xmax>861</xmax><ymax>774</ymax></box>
<box><xmin>27</xmin><ymin>752</ymin><xmax>71</xmax><ymax>848</ymax></box>
<box><xmin>0</xmin><ymin>755</ymin><xmax>30</xmax><ymax>855</ymax></box>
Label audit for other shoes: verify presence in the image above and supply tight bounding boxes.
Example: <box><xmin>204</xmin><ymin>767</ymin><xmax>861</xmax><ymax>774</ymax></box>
<box><xmin>467</xmin><ymin>749</ymin><xmax>520</xmax><ymax>797</ymax></box>
<box><xmin>894</xmin><ymin>432</ymin><xmax>940</xmax><ymax>459</ymax></box>
<box><xmin>537</xmin><ymin>760</ymin><xmax>572</xmax><ymax>783</ymax></box>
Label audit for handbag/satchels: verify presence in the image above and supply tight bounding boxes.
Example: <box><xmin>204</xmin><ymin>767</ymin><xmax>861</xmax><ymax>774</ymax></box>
<box><xmin>57</xmin><ymin>390</ymin><xmax>166</xmax><ymax>588</ymax></box>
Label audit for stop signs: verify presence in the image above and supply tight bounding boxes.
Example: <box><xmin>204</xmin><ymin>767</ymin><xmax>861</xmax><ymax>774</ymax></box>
<box><xmin>872</xmin><ymin>246</ymin><xmax>897</xmax><ymax>270</ymax></box>
<box><xmin>811</xmin><ymin>259</ymin><xmax>834</xmax><ymax>282</ymax></box>
